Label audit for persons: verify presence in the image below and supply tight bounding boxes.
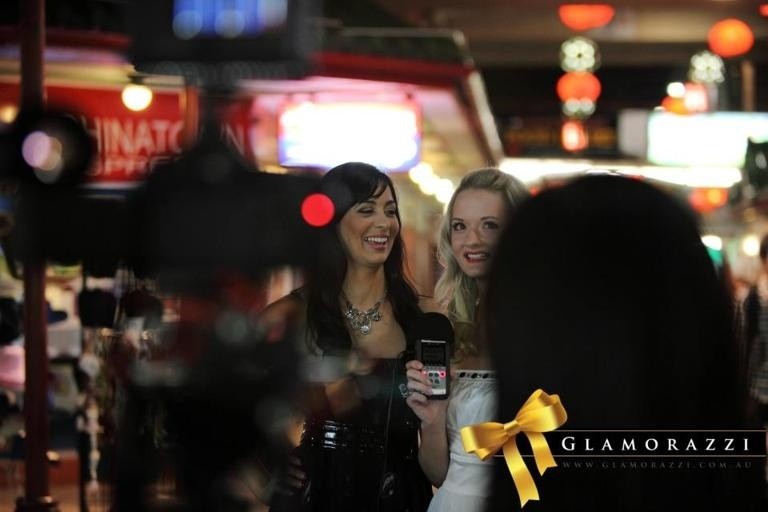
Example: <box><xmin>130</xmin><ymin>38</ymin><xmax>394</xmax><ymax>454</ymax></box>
<box><xmin>404</xmin><ymin>167</ymin><xmax>532</xmax><ymax>512</ymax></box>
<box><xmin>738</xmin><ymin>234</ymin><xmax>768</xmax><ymax>430</ymax></box>
<box><xmin>480</xmin><ymin>171</ymin><xmax>768</xmax><ymax>512</ymax></box>
<box><xmin>250</xmin><ymin>161</ymin><xmax>436</xmax><ymax>512</ymax></box>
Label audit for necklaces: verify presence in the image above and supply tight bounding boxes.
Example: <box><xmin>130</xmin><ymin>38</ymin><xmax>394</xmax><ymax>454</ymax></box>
<box><xmin>339</xmin><ymin>289</ymin><xmax>389</xmax><ymax>335</ymax></box>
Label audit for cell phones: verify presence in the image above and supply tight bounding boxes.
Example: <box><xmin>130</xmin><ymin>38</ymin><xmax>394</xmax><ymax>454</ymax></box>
<box><xmin>415</xmin><ymin>339</ymin><xmax>451</xmax><ymax>400</ymax></box>
